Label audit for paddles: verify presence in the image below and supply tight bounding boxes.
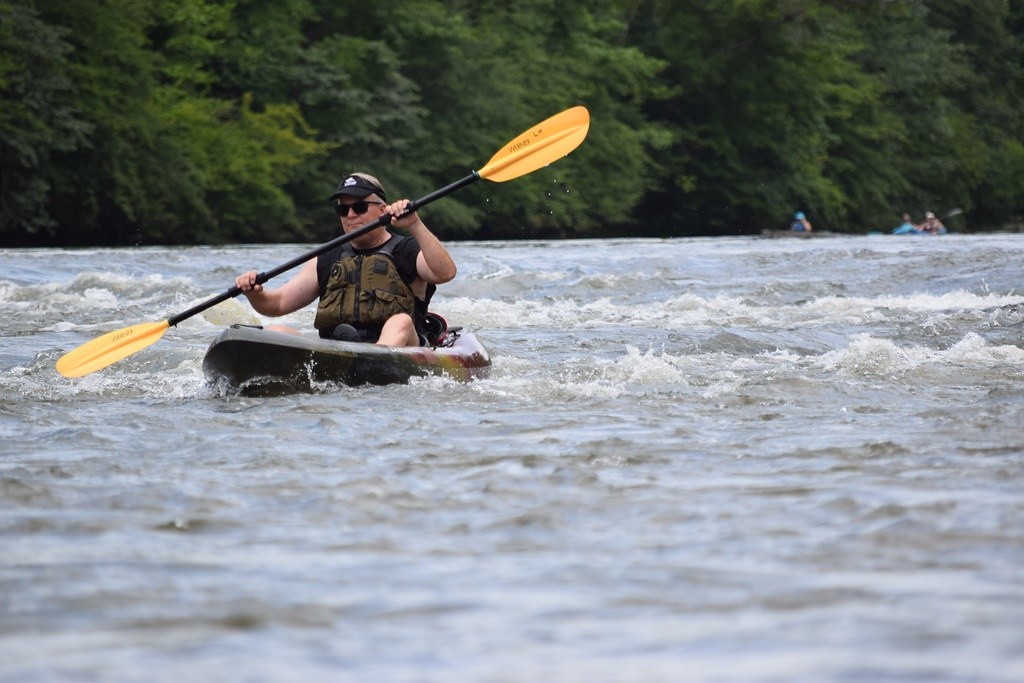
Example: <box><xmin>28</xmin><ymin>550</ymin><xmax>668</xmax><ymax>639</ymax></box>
<box><xmin>921</xmin><ymin>210</ymin><xmax>961</xmax><ymax>228</ymax></box>
<box><xmin>56</xmin><ymin>105</ymin><xmax>590</xmax><ymax>379</ymax></box>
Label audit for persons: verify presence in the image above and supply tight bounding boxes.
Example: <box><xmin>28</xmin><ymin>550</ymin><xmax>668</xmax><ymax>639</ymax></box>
<box><xmin>904</xmin><ymin>214</ymin><xmax>919</xmax><ymax>230</ymax></box>
<box><xmin>920</xmin><ymin>215</ymin><xmax>937</xmax><ymax>232</ymax></box>
<box><xmin>235</xmin><ymin>173</ymin><xmax>457</xmax><ymax>346</ymax></box>
<box><xmin>791</xmin><ymin>211</ymin><xmax>811</xmax><ymax>232</ymax></box>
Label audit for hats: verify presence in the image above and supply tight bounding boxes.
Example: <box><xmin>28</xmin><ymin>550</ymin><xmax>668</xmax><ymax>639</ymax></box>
<box><xmin>328</xmin><ymin>176</ymin><xmax>386</xmax><ymax>202</ymax></box>
<box><xmin>794</xmin><ymin>211</ymin><xmax>805</xmax><ymax>221</ymax></box>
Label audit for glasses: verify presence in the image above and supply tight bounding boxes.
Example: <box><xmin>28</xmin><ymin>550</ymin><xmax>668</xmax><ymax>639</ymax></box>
<box><xmin>335</xmin><ymin>201</ymin><xmax>382</xmax><ymax>217</ymax></box>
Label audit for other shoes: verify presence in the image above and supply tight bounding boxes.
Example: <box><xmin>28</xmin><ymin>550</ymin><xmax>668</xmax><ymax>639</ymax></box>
<box><xmin>331</xmin><ymin>324</ymin><xmax>362</xmax><ymax>342</ymax></box>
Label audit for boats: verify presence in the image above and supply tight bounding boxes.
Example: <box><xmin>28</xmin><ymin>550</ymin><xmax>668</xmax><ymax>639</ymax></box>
<box><xmin>762</xmin><ymin>229</ymin><xmax>835</xmax><ymax>239</ymax></box>
<box><xmin>893</xmin><ymin>223</ymin><xmax>947</xmax><ymax>235</ymax></box>
<box><xmin>201</xmin><ymin>321</ymin><xmax>494</xmax><ymax>398</ymax></box>
